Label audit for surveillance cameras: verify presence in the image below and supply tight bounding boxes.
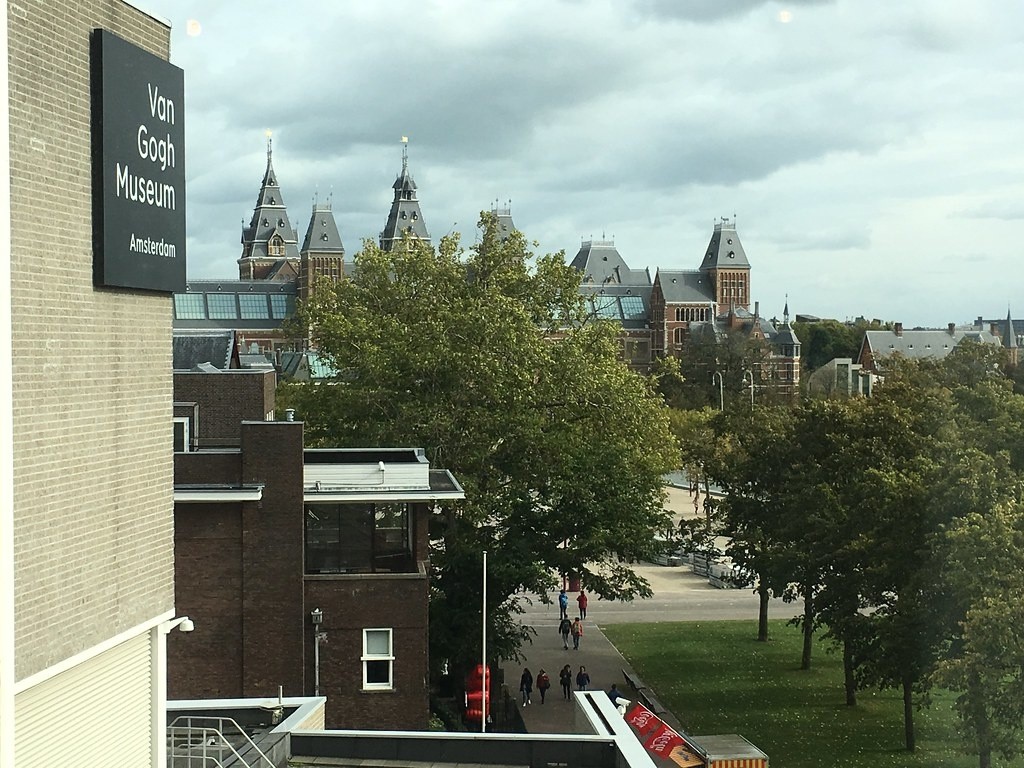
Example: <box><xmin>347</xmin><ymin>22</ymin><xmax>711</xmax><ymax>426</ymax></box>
<box><xmin>179</xmin><ymin>616</ymin><xmax>195</xmax><ymax>634</ymax></box>
<box><xmin>616</xmin><ymin>697</ymin><xmax>631</xmax><ymax>717</ymax></box>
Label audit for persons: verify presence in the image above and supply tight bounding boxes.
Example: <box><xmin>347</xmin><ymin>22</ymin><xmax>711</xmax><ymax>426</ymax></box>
<box><xmin>558</xmin><ymin>590</ymin><xmax>568</xmax><ymax>620</ymax></box>
<box><xmin>560</xmin><ymin>664</ymin><xmax>590</xmax><ymax>700</ymax></box>
<box><xmin>576</xmin><ymin>591</ymin><xmax>587</xmax><ymax>621</ymax></box>
<box><xmin>559</xmin><ymin>614</ymin><xmax>583</xmax><ymax>650</ymax></box>
<box><xmin>518</xmin><ymin>668</ymin><xmax>550</xmax><ymax>707</ymax></box>
<box><xmin>607</xmin><ymin>683</ymin><xmax>624</xmax><ymax>708</ymax></box>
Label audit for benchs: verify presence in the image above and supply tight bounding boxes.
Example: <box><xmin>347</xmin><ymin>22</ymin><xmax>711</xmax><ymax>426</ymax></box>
<box><xmin>621</xmin><ymin>667</ymin><xmax>646</xmax><ymax>697</ymax></box>
<box><xmin>639</xmin><ymin>690</ymin><xmax>668</xmax><ymax>717</ymax></box>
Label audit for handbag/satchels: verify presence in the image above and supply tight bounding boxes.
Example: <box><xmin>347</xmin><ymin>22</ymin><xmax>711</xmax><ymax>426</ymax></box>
<box><xmin>544</xmin><ymin>680</ymin><xmax>550</xmax><ymax>689</ymax></box>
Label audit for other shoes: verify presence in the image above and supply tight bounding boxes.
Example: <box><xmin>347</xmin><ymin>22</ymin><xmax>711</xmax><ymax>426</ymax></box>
<box><xmin>523</xmin><ymin>703</ymin><xmax>526</xmax><ymax>707</ymax></box>
<box><xmin>564</xmin><ymin>646</ymin><xmax>568</xmax><ymax>650</ymax></box>
<box><xmin>573</xmin><ymin>647</ymin><xmax>579</xmax><ymax>650</ymax></box>
<box><xmin>528</xmin><ymin>700</ymin><xmax>531</xmax><ymax>704</ymax></box>
<box><xmin>559</xmin><ymin>618</ymin><xmax>563</xmax><ymax>620</ymax></box>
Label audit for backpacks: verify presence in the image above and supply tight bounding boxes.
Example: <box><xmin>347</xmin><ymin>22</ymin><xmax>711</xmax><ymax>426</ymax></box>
<box><xmin>563</xmin><ymin>619</ymin><xmax>570</xmax><ymax>632</ymax></box>
<box><xmin>560</xmin><ymin>594</ymin><xmax>566</xmax><ymax>605</ymax></box>
<box><xmin>574</xmin><ymin>622</ymin><xmax>580</xmax><ymax>634</ymax></box>
